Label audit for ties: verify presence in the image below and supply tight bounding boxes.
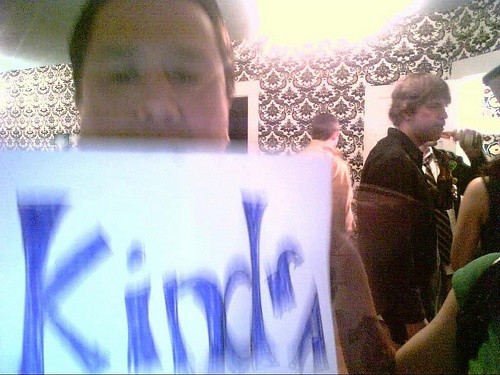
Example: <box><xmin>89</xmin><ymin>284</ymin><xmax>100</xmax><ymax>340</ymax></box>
<box><xmin>422</xmin><ymin>155</ymin><xmax>454</xmax><ymax>264</ymax></box>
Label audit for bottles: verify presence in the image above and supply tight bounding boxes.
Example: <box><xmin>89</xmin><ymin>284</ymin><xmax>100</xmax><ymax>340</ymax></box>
<box><xmin>436</xmin><ymin>157</ymin><xmax>452</xmax><ymax>211</ymax></box>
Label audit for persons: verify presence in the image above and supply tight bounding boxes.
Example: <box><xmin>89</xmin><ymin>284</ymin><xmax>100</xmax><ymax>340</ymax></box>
<box><xmin>300</xmin><ymin>68</ymin><xmax>500</xmax><ymax>375</ymax></box>
<box><xmin>68</xmin><ymin>0</ymin><xmax>238</xmax><ymax>153</ymax></box>
